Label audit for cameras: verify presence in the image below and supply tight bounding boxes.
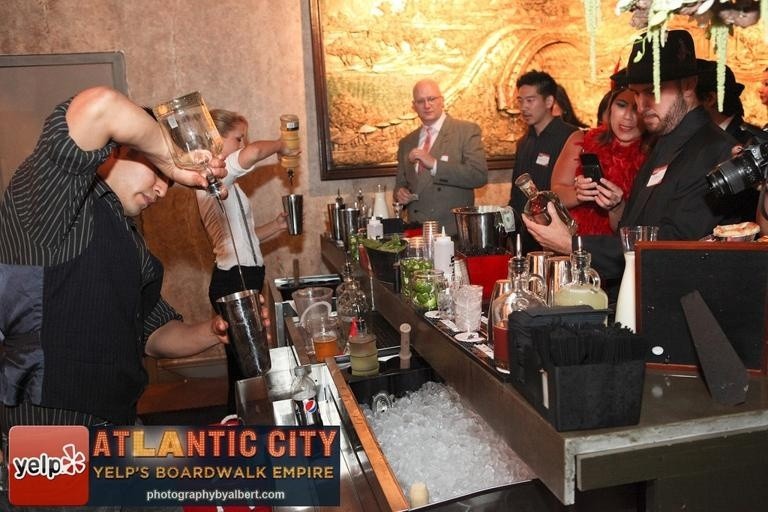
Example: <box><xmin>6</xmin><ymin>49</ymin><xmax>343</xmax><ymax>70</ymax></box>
<box><xmin>706</xmin><ymin>124</ymin><xmax>768</xmax><ymax>199</ymax></box>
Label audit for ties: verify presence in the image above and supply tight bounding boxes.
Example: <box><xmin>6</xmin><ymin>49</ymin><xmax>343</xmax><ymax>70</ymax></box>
<box><xmin>418</xmin><ymin>128</ymin><xmax>431</xmax><ymax>174</ymax></box>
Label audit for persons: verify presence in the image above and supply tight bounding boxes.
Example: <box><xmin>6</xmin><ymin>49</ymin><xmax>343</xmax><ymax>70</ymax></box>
<box><xmin>694</xmin><ymin>58</ymin><xmax>757</xmax><ymax>145</ymax></box>
<box><xmin>732</xmin><ymin>146</ymin><xmax>768</xmax><ymax>238</ymax></box>
<box><xmin>508</xmin><ymin>70</ymin><xmax>580</xmax><ymax>253</ymax></box>
<box><xmin>393</xmin><ymin>79</ymin><xmax>489</xmax><ymax>236</ymax></box>
<box><xmin>189</xmin><ymin>109</ymin><xmax>302</xmax><ymax>422</ymax></box>
<box><xmin>550</xmin><ymin>69</ymin><xmax>651</xmax><ymax>236</ymax></box>
<box><xmin>0</xmin><ymin>86</ymin><xmax>272</xmax><ymax>429</ymax></box>
<box><xmin>759</xmin><ymin>67</ymin><xmax>768</xmax><ymax>104</ymax></box>
<box><xmin>522</xmin><ymin>29</ymin><xmax>742</xmax><ymax>294</ymax></box>
<box><xmin>552</xmin><ymin>84</ymin><xmax>590</xmax><ymax>128</ymax></box>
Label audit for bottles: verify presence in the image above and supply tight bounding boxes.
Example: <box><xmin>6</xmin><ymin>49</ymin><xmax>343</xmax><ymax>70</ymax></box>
<box><xmin>349</xmin><ymin>319</ymin><xmax>380</xmax><ymax>376</ymax></box>
<box><xmin>491</xmin><ymin>173</ymin><xmax>635</xmax><ymax>376</ymax></box>
<box><xmin>152</xmin><ymin>91</ymin><xmax>299</xmax><ymax>191</ymax></box>
<box><xmin>353</xmin><ymin>189</ymin><xmax>368</xmax><ymax>219</ymax></box>
<box><xmin>290</xmin><ymin>365</ymin><xmax>323</xmax><ymax>427</ymax></box>
<box><xmin>434</xmin><ymin>226</ymin><xmax>454</xmax><ymax>277</ymax></box>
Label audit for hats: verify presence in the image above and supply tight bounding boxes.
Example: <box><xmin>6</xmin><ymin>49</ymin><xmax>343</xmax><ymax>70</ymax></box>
<box><xmin>698</xmin><ymin>61</ymin><xmax>745</xmax><ymax>96</ymax></box>
<box><xmin>610</xmin><ymin>30</ymin><xmax>716</xmax><ymax>83</ymax></box>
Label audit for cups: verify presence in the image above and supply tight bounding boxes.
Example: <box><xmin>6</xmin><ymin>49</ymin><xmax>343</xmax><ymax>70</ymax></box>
<box><xmin>281</xmin><ymin>194</ymin><xmax>360</xmax><ymax>249</ymax></box>
<box><xmin>291</xmin><ymin>287</ymin><xmax>341</xmax><ymax>363</ymax></box>
<box><xmin>486</xmin><ymin>279</ymin><xmax>511</xmax><ymax>343</ymax></box>
<box><xmin>400</xmin><ymin>257</ymin><xmax>483</xmax><ymax>332</ymax></box>
<box><xmin>619</xmin><ymin>226</ymin><xmax>659</xmax><ymax>251</ymax></box>
<box><xmin>409</xmin><ymin>221</ymin><xmax>439</xmax><ymax>258</ymax></box>
<box><xmin>215</xmin><ymin>289</ymin><xmax>271</xmax><ymax>378</ymax></box>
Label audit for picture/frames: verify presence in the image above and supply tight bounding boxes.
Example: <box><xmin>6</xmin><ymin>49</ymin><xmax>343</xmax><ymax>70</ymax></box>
<box><xmin>307</xmin><ymin>0</ymin><xmax>768</xmax><ymax>180</ymax></box>
<box><xmin>635</xmin><ymin>240</ymin><xmax>768</xmax><ymax>404</ymax></box>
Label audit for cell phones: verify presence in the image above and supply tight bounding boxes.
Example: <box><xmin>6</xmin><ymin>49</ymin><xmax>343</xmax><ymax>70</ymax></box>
<box><xmin>579</xmin><ymin>154</ymin><xmax>603</xmax><ymax>185</ymax></box>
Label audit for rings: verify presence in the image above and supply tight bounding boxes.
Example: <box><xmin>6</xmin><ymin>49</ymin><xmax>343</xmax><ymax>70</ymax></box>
<box><xmin>574</xmin><ymin>175</ymin><xmax>583</xmax><ymax>183</ymax></box>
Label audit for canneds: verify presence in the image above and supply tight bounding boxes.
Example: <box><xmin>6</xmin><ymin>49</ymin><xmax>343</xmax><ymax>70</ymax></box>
<box><xmin>399</xmin><ymin>256</ymin><xmax>434</xmax><ymax>303</ymax></box>
<box><xmin>409</xmin><ymin>269</ymin><xmax>448</xmax><ymax>312</ymax></box>
